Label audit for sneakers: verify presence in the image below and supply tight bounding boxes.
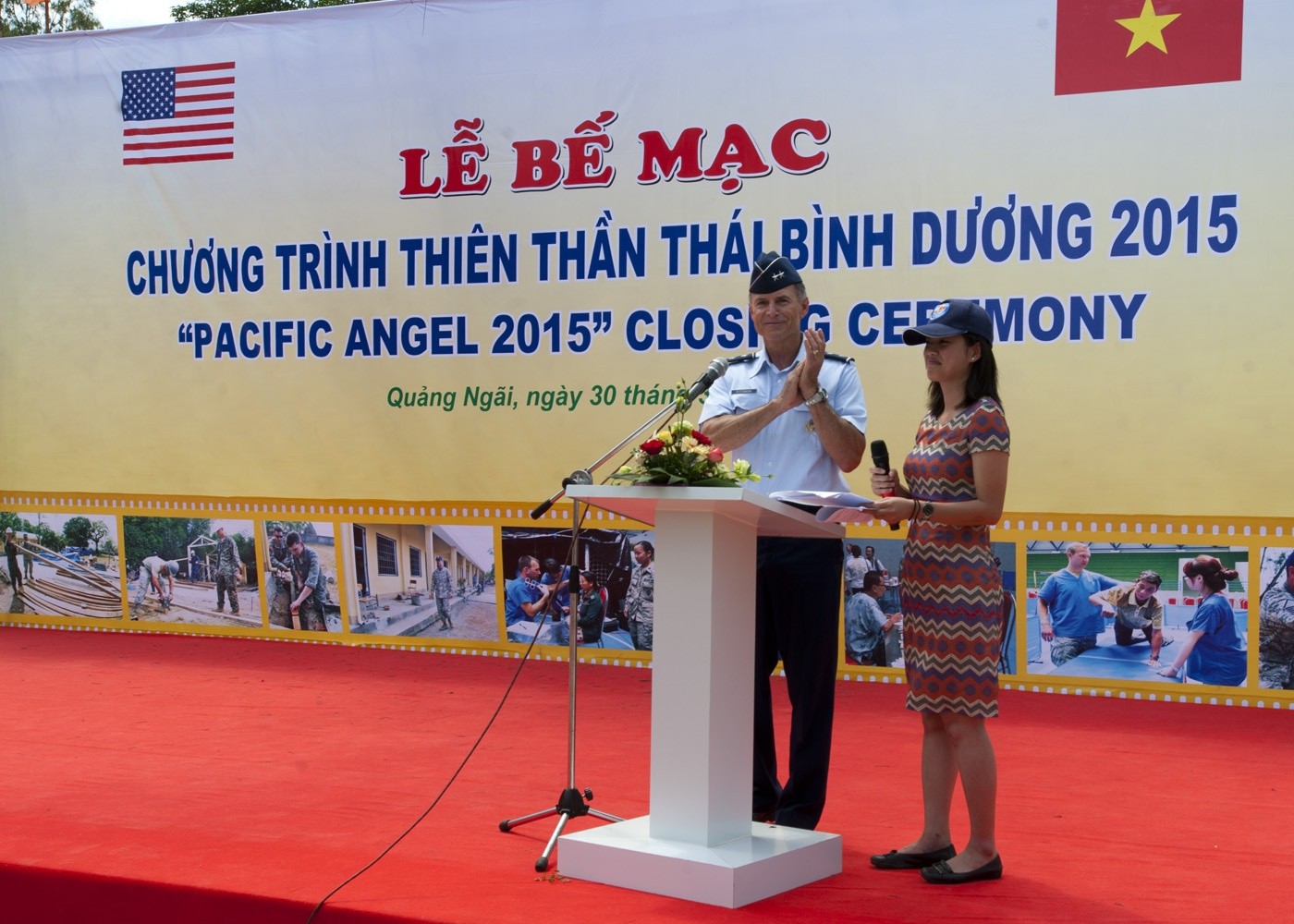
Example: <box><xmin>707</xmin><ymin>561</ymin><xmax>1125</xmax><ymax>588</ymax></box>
<box><xmin>440</xmin><ymin>624</ymin><xmax>448</xmax><ymax>630</ymax></box>
<box><xmin>449</xmin><ymin>621</ymin><xmax>453</xmax><ymax>628</ymax></box>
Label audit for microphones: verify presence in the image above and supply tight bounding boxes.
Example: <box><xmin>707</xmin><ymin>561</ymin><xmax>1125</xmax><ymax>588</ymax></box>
<box><xmin>871</xmin><ymin>439</ymin><xmax>901</xmax><ymax>530</ymax></box>
<box><xmin>675</xmin><ymin>358</ymin><xmax>730</xmax><ymax>412</ymax></box>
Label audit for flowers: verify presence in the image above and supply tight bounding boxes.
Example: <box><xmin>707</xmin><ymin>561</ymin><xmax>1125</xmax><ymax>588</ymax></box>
<box><xmin>603</xmin><ymin>377</ymin><xmax>776</xmax><ymax>488</ymax></box>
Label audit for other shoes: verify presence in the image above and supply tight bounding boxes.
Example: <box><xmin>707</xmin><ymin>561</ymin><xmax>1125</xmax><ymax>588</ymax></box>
<box><xmin>870</xmin><ymin>843</ymin><xmax>957</xmax><ymax>869</ymax></box>
<box><xmin>921</xmin><ymin>853</ymin><xmax>1003</xmax><ymax>883</ymax></box>
<box><xmin>232</xmin><ymin>610</ymin><xmax>240</xmax><ymax>616</ymax></box>
<box><xmin>212</xmin><ymin>607</ymin><xmax>224</xmax><ymax>612</ymax></box>
<box><xmin>134</xmin><ymin>603</ymin><xmax>145</xmax><ymax>614</ymax></box>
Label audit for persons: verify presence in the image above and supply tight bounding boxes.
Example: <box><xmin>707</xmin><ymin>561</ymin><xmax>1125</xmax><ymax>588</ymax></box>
<box><xmin>868</xmin><ymin>299</ymin><xmax>1011</xmax><ymax>884</ymax></box>
<box><xmin>430</xmin><ymin>556</ymin><xmax>484</xmax><ymax>631</ymax></box>
<box><xmin>505</xmin><ymin>541</ymin><xmax>654</xmax><ymax>651</ymax></box>
<box><xmin>1158</xmin><ymin>555</ymin><xmax>1247</xmax><ymax>687</ymax></box>
<box><xmin>1259</xmin><ymin>552</ymin><xmax>1294</xmax><ymax>685</ymax></box>
<box><xmin>130</xmin><ymin>526</ymin><xmax>242</xmax><ymax>620</ymax></box>
<box><xmin>4</xmin><ymin>527</ymin><xmax>34</xmax><ymax>596</ymax></box>
<box><xmin>844</xmin><ymin>544</ymin><xmax>901</xmax><ymax>667</ymax></box>
<box><xmin>1089</xmin><ymin>570</ymin><xmax>1175</xmax><ymax>669</ymax></box>
<box><xmin>269</xmin><ymin>527</ymin><xmax>327</xmax><ymax>612</ymax></box>
<box><xmin>698</xmin><ymin>251</ymin><xmax>868</xmax><ymax>831</ymax></box>
<box><xmin>1038</xmin><ymin>542</ymin><xmax>1134</xmax><ymax>668</ymax></box>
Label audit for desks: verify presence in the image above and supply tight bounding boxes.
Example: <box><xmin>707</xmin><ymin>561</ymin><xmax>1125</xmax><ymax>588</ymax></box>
<box><xmin>507</xmin><ymin>620</ymin><xmax>553</xmax><ymax>644</ymax></box>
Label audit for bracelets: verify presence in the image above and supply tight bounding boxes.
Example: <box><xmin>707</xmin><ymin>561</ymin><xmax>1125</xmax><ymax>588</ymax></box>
<box><xmin>908</xmin><ymin>499</ymin><xmax>921</xmax><ymax>523</ymax></box>
<box><xmin>1171</xmin><ymin>666</ymin><xmax>1179</xmax><ymax>672</ymax></box>
<box><xmin>1150</xmin><ymin>654</ymin><xmax>1159</xmax><ymax>660</ymax></box>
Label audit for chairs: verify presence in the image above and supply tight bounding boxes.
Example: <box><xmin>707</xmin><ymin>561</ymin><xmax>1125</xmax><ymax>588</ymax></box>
<box><xmin>356</xmin><ymin>583</ymin><xmax>377</xmax><ymax>620</ymax></box>
<box><xmin>997</xmin><ymin>591</ymin><xmax>1016</xmax><ymax>675</ymax></box>
<box><xmin>1169</xmin><ymin>597</ymin><xmax>1248</xmax><ymax>609</ymax></box>
<box><xmin>406</xmin><ymin>579</ymin><xmax>417</xmax><ymax>598</ymax></box>
<box><xmin>576</xmin><ymin>586</ymin><xmax>609</xmax><ymax>648</ymax></box>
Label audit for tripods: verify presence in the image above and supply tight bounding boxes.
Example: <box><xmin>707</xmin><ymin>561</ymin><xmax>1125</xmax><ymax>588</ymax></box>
<box><xmin>499</xmin><ymin>399</ymin><xmax>677</xmax><ymax>873</ymax></box>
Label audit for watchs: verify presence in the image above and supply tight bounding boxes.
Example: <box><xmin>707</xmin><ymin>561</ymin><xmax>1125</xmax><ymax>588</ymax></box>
<box><xmin>921</xmin><ymin>501</ymin><xmax>935</xmax><ymax>521</ymax></box>
<box><xmin>804</xmin><ymin>386</ymin><xmax>828</xmax><ymax>407</ymax></box>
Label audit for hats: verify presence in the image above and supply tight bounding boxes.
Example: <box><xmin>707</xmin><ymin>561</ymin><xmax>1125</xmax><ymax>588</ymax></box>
<box><xmin>5</xmin><ymin>527</ymin><xmax>13</xmax><ymax>534</ymax></box>
<box><xmin>435</xmin><ymin>555</ymin><xmax>442</xmax><ymax>560</ymax></box>
<box><xmin>169</xmin><ymin>560</ymin><xmax>180</xmax><ymax>576</ymax></box>
<box><xmin>211</xmin><ymin>524</ymin><xmax>223</xmax><ymax>532</ymax></box>
<box><xmin>749</xmin><ymin>251</ymin><xmax>803</xmax><ymax>294</ymax></box>
<box><xmin>902</xmin><ymin>299</ymin><xmax>993</xmax><ymax>348</ymax></box>
